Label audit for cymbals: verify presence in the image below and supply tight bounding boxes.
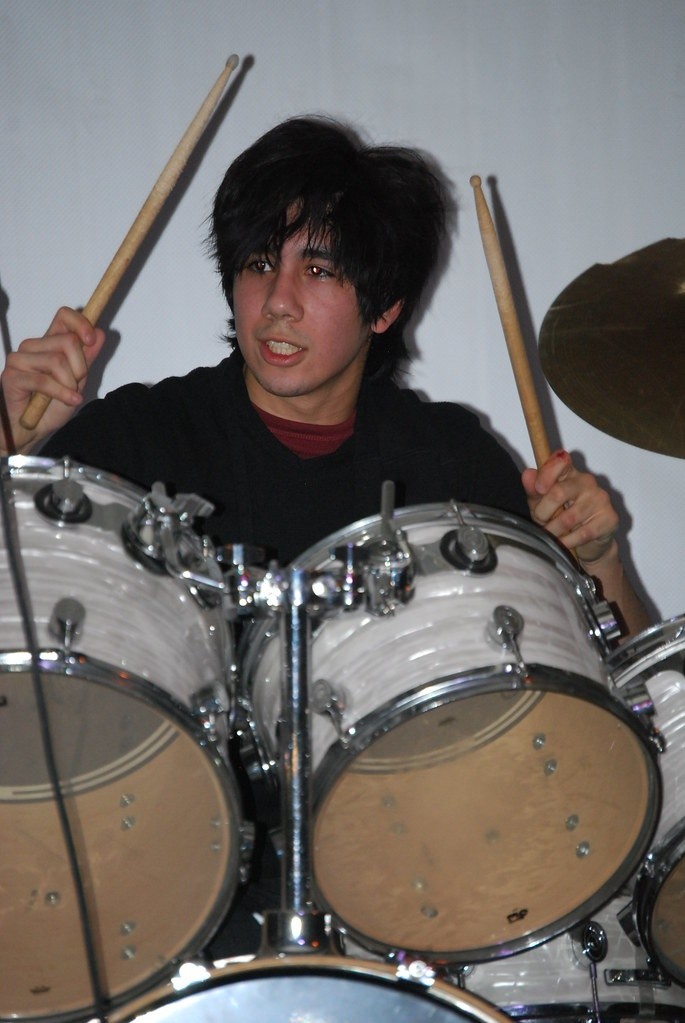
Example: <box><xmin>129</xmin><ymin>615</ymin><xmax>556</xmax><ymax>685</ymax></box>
<box><xmin>537</xmin><ymin>237</ymin><xmax>685</xmax><ymax>461</ymax></box>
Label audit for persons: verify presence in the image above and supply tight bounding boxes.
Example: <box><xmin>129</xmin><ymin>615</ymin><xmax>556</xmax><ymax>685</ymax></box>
<box><xmin>1</xmin><ymin>118</ymin><xmax>649</xmax><ymax>964</ymax></box>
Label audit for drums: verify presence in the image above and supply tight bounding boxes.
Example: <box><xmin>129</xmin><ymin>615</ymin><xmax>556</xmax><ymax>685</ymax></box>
<box><xmin>237</xmin><ymin>497</ymin><xmax>666</xmax><ymax>966</ymax></box>
<box><xmin>92</xmin><ymin>957</ymin><xmax>515</xmax><ymax>1023</ymax></box>
<box><xmin>429</xmin><ymin>890</ymin><xmax>685</xmax><ymax>1016</ymax></box>
<box><xmin>607</xmin><ymin>611</ymin><xmax>685</xmax><ymax>991</ymax></box>
<box><xmin>0</xmin><ymin>452</ymin><xmax>245</xmax><ymax>1016</ymax></box>
